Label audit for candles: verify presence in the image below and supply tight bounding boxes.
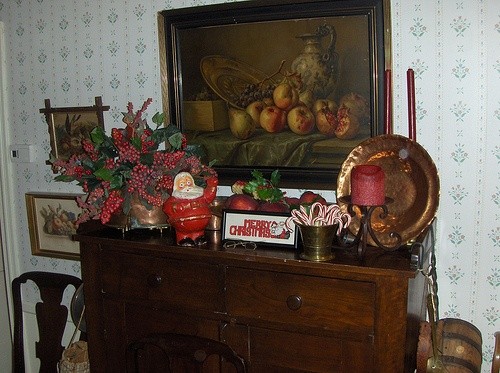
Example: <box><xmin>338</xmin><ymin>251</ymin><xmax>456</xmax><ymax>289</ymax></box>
<box><xmin>406</xmin><ymin>68</ymin><xmax>416</xmax><ymax>143</ymax></box>
<box><xmin>351</xmin><ymin>164</ymin><xmax>386</xmax><ymax>205</ymax></box>
<box><xmin>384</xmin><ymin>69</ymin><xmax>392</xmax><ymax>134</ymax></box>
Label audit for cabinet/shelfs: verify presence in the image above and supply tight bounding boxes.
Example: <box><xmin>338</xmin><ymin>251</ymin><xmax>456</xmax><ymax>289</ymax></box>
<box><xmin>69</xmin><ymin>217</ymin><xmax>438</xmax><ymax>373</ymax></box>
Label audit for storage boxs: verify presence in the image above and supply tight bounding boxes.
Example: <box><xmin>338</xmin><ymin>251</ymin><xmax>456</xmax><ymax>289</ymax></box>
<box><xmin>182</xmin><ymin>99</ymin><xmax>231</xmax><ymax>132</ymax></box>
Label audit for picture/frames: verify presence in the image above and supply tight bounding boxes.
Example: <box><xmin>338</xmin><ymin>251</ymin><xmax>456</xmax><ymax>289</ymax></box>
<box><xmin>39</xmin><ymin>95</ymin><xmax>110</xmax><ymax>174</ymax></box>
<box><xmin>156</xmin><ymin>0</ymin><xmax>394</xmax><ymax>190</ymax></box>
<box><xmin>25</xmin><ymin>192</ymin><xmax>86</xmax><ymax>261</ymax></box>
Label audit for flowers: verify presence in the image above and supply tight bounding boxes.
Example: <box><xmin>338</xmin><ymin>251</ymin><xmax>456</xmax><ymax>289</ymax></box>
<box><xmin>44</xmin><ymin>97</ymin><xmax>216</xmax><ymax>231</ymax></box>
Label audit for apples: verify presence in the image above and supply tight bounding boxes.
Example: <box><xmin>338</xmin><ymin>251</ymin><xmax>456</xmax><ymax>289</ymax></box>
<box><xmin>226</xmin><ymin>190</ymin><xmax>327</xmax><ymax>215</ymax></box>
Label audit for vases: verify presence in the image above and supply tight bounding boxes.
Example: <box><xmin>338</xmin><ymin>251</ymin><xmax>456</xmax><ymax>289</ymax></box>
<box><xmin>99</xmin><ymin>182</ymin><xmax>172</xmax><ymax>236</ymax></box>
<box><xmin>290</xmin><ymin>23</ymin><xmax>339</xmax><ymax>101</ymax></box>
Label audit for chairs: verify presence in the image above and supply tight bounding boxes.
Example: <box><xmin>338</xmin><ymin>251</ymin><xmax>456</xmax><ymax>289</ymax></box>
<box><xmin>11</xmin><ymin>271</ymin><xmax>88</xmax><ymax>373</ymax></box>
<box><xmin>125</xmin><ymin>334</ymin><xmax>249</xmax><ymax>373</ymax></box>
